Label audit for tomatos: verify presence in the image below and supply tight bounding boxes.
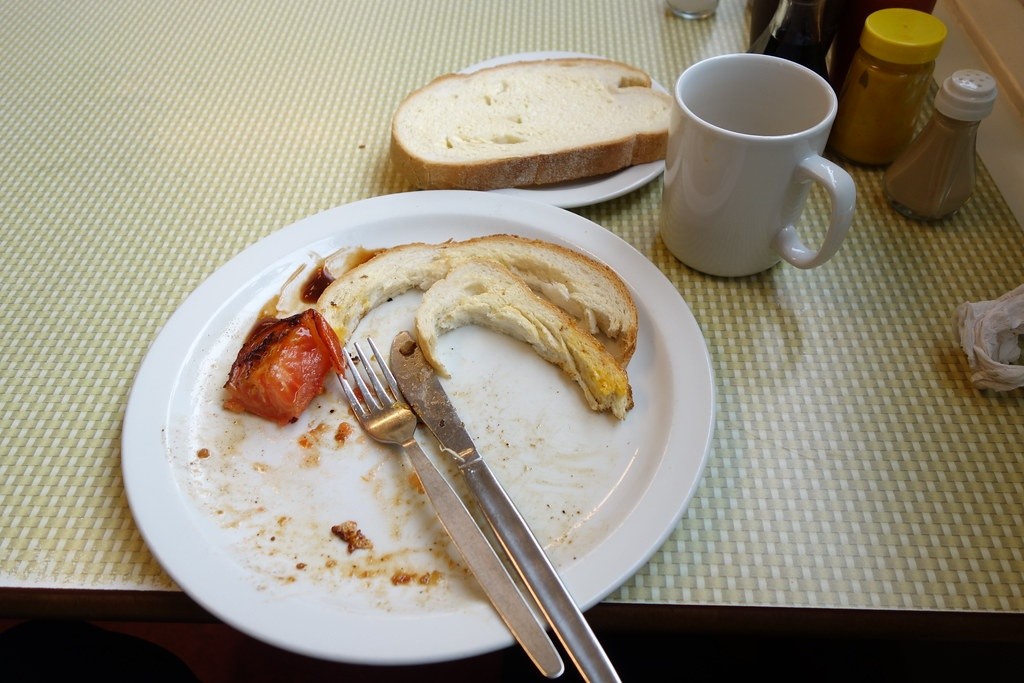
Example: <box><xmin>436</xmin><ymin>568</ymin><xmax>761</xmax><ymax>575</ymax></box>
<box><xmin>223</xmin><ymin>310</ymin><xmax>346</xmax><ymax>428</ymax></box>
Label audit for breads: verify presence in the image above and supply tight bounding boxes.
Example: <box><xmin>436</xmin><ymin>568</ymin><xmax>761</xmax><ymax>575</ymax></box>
<box><xmin>314</xmin><ymin>234</ymin><xmax>639</xmax><ymax>420</ymax></box>
<box><xmin>391</xmin><ymin>59</ymin><xmax>671</xmax><ymax>191</ymax></box>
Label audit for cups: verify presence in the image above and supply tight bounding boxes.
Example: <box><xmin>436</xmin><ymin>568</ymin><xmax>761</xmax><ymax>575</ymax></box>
<box><xmin>657</xmin><ymin>50</ymin><xmax>857</xmax><ymax>279</ymax></box>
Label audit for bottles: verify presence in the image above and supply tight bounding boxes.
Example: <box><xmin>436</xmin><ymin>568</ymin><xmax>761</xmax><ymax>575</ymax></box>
<box><xmin>825</xmin><ymin>8</ymin><xmax>946</xmax><ymax>171</ymax></box>
<box><xmin>880</xmin><ymin>67</ymin><xmax>1000</xmax><ymax>224</ymax></box>
<box><xmin>746</xmin><ymin>0</ymin><xmax>823</xmax><ymax>94</ymax></box>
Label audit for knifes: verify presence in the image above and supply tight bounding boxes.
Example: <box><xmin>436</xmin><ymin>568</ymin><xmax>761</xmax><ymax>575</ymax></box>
<box><xmin>391</xmin><ymin>323</ymin><xmax>620</xmax><ymax>681</ymax></box>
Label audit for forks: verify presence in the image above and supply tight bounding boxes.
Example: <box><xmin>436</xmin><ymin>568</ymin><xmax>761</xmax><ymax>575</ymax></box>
<box><xmin>330</xmin><ymin>336</ymin><xmax>565</xmax><ymax>683</ymax></box>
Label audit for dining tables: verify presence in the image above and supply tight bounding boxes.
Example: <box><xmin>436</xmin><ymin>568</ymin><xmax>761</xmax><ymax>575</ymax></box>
<box><xmin>0</xmin><ymin>1</ymin><xmax>1023</xmax><ymax>653</ymax></box>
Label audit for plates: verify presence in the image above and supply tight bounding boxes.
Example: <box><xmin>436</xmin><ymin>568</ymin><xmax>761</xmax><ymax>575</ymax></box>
<box><xmin>435</xmin><ymin>48</ymin><xmax>676</xmax><ymax>211</ymax></box>
<box><xmin>118</xmin><ymin>189</ymin><xmax>717</xmax><ymax>663</ymax></box>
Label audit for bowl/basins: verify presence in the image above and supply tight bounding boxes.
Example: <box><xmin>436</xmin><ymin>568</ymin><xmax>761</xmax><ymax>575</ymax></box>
<box><xmin>666</xmin><ymin>0</ymin><xmax>720</xmax><ymax>20</ymax></box>
